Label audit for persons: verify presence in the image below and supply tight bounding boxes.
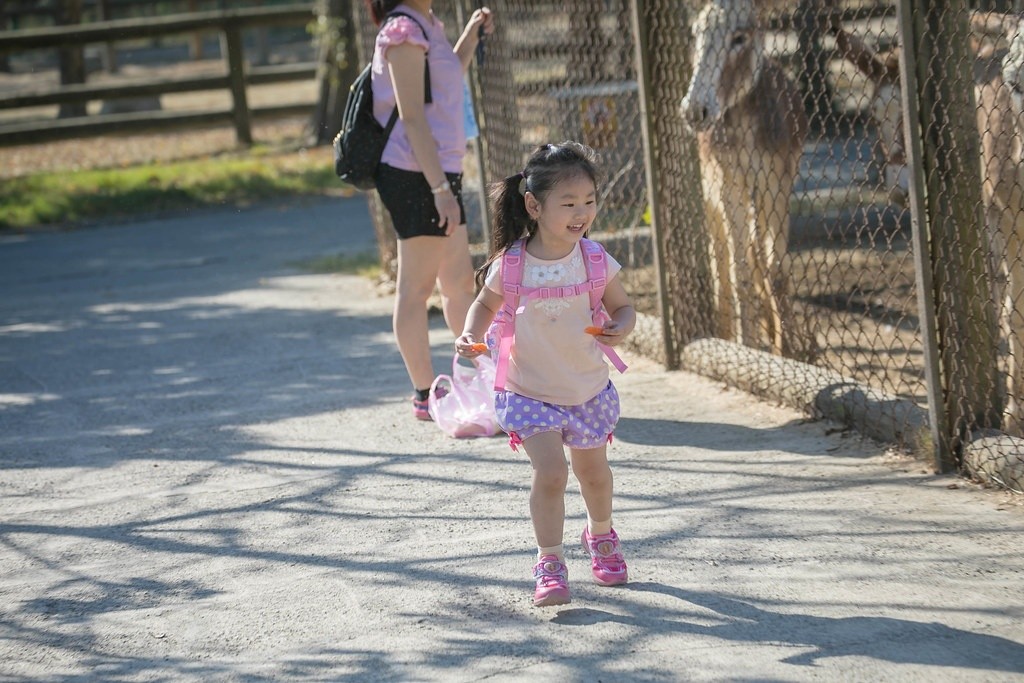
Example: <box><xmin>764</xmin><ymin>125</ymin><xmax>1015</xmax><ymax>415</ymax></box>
<box><xmin>371</xmin><ymin>0</ymin><xmax>497</xmax><ymax>421</ymax></box>
<box><xmin>455</xmin><ymin>139</ymin><xmax>636</xmax><ymax>606</ymax></box>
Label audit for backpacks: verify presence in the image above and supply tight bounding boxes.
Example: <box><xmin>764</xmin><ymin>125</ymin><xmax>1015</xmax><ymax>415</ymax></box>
<box><xmin>333</xmin><ymin>11</ymin><xmax>432</xmax><ymax>190</ymax></box>
<box><xmin>485</xmin><ymin>237</ymin><xmax>608</xmax><ymax>367</ymax></box>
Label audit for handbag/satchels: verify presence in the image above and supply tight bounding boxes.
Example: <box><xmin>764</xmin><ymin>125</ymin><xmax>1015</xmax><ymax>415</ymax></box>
<box><xmin>428</xmin><ymin>350</ymin><xmax>505</xmax><ymax>438</ymax></box>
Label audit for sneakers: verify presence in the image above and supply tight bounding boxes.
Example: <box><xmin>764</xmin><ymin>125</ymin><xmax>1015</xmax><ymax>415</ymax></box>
<box><xmin>581</xmin><ymin>525</ymin><xmax>627</xmax><ymax>586</ymax></box>
<box><xmin>411</xmin><ymin>387</ymin><xmax>450</xmax><ymax>420</ymax></box>
<box><xmin>532</xmin><ymin>554</ymin><xmax>571</xmax><ymax>607</ymax></box>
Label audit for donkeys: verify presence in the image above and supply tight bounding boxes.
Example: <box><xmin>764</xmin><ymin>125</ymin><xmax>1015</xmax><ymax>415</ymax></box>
<box><xmin>829</xmin><ymin>0</ymin><xmax>1024</xmax><ymax>441</ymax></box>
<box><xmin>677</xmin><ymin>2</ymin><xmax>809</xmax><ymax>360</ymax></box>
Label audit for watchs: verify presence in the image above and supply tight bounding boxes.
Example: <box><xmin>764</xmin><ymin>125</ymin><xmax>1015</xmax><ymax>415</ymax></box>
<box><xmin>431</xmin><ymin>181</ymin><xmax>450</xmax><ymax>194</ymax></box>
